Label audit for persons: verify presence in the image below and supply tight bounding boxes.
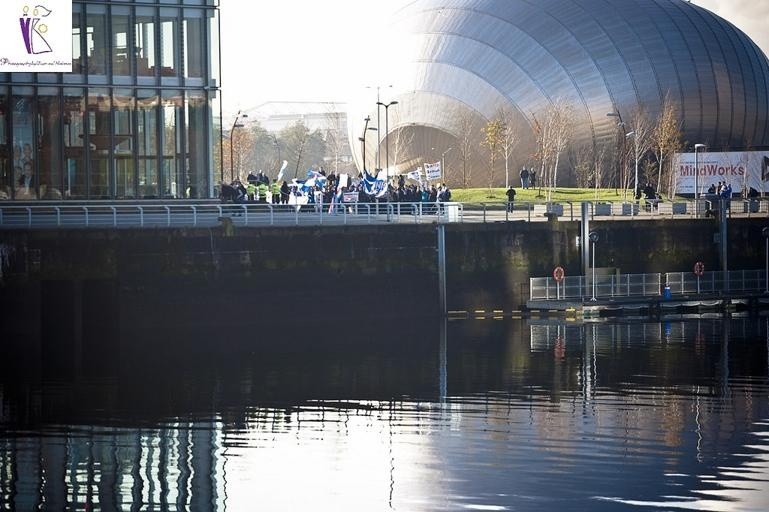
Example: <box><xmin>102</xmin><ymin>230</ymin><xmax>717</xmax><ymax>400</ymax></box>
<box><xmin>506</xmin><ymin>186</ymin><xmax>516</xmax><ymax>213</ymax></box>
<box><xmin>13</xmin><ymin>143</ymin><xmax>34</xmax><ymax>195</ymax></box>
<box><xmin>218</xmin><ymin>167</ymin><xmax>451</xmax><ymax>217</ymax></box>
<box><xmin>633</xmin><ymin>182</ymin><xmax>664</xmax><ymax>212</ymax></box>
<box><xmin>708</xmin><ymin>181</ymin><xmax>732</xmax><ymax>218</ymax></box>
<box><xmin>519</xmin><ymin>165</ymin><xmax>536</xmax><ymax>189</ymax></box>
<box><xmin>748</xmin><ymin>187</ymin><xmax>758</xmax><ymax>200</ymax></box>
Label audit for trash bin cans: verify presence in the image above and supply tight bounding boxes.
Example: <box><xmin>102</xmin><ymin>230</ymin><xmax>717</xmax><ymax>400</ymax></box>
<box><xmin>448</xmin><ymin>203</ymin><xmax>458</xmax><ymax>223</ymax></box>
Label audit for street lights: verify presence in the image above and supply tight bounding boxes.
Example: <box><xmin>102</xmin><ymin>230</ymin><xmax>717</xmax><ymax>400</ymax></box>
<box><xmin>273</xmin><ymin>134</ymin><xmax>281</xmax><ymax>180</ymax></box>
<box><xmin>376</xmin><ymin>100</ymin><xmax>400</xmax><ymax>189</ymax></box>
<box><xmin>231</xmin><ymin>109</ymin><xmax>247</xmax><ymax>182</ymax></box>
<box><xmin>693</xmin><ymin>142</ymin><xmax>707</xmax><ymax>217</ymax></box>
<box><xmin>587</xmin><ymin>231</ymin><xmax>599</xmax><ymax>303</ymax></box>
<box><xmin>365</xmin><ymin>84</ymin><xmax>392</xmax><ymax>168</ymax></box>
<box><xmin>606</xmin><ymin>106</ymin><xmax>627</xmax><ymax>153</ymax></box>
<box><xmin>359</xmin><ymin>115</ymin><xmax>379</xmax><ymax>171</ymax></box>
<box><xmin>430</xmin><ymin>146</ymin><xmax>454</xmax><ymax>184</ymax></box>
<box><xmin>624</xmin><ymin>130</ymin><xmax>638</xmax><ymax>198</ymax></box>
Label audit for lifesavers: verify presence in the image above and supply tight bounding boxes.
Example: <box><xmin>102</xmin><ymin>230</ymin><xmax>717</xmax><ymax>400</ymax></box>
<box><xmin>554</xmin><ymin>267</ymin><xmax>565</xmax><ymax>281</ymax></box>
<box><xmin>694</xmin><ymin>262</ymin><xmax>704</xmax><ymax>275</ymax></box>
